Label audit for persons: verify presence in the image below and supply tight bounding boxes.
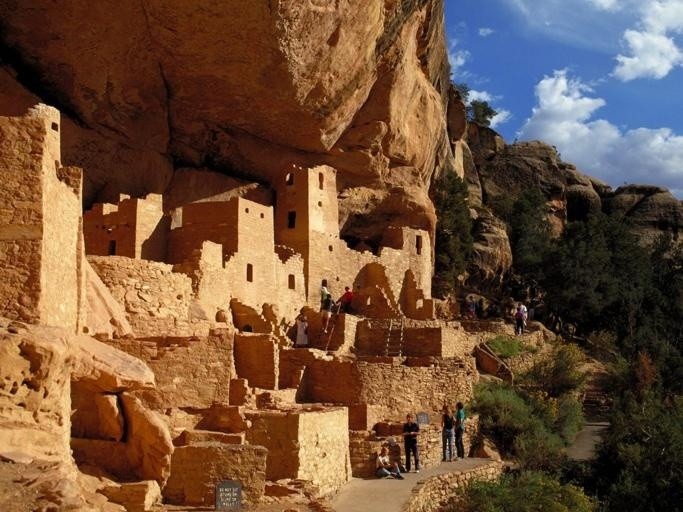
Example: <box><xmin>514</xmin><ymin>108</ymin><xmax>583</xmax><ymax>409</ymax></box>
<box><xmin>467</xmin><ymin>296</ymin><xmax>474</xmax><ymax>319</ymax></box>
<box><xmin>320</xmin><ymin>293</ymin><xmax>340</xmax><ymax>334</ymax></box>
<box><xmin>517</xmin><ymin>301</ymin><xmax>527</xmax><ymax>328</ymax></box>
<box><xmin>440</xmin><ymin>403</ymin><xmax>456</xmax><ymax>463</ymax></box>
<box><xmin>475</xmin><ymin>296</ymin><xmax>483</xmax><ymax>321</ymax></box>
<box><xmin>514</xmin><ymin>307</ymin><xmax>523</xmax><ymax>336</ymax></box>
<box><xmin>320</xmin><ymin>279</ymin><xmax>330</xmax><ymax>312</ymax></box>
<box><xmin>373</xmin><ymin>446</ymin><xmax>404</xmax><ymax>480</ymax></box>
<box><xmin>400</xmin><ymin>412</ymin><xmax>420</xmax><ymax>473</ymax></box>
<box><xmin>335</xmin><ymin>285</ymin><xmax>353</xmax><ymax>315</ymax></box>
<box><xmin>294</xmin><ymin>313</ymin><xmax>308</xmax><ymax>348</ymax></box>
<box><xmin>453</xmin><ymin>401</ymin><xmax>465</xmax><ymax>462</ymax></box>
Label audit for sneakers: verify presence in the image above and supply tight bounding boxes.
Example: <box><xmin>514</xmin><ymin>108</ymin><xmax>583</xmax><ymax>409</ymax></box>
<box><xmin>392</xmin><ymin>468</ymin><xmax>420</xmax><ymax>480</ymax></box>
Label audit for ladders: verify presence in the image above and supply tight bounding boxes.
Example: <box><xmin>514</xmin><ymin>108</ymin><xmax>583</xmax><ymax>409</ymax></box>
<box><xmin>385</xmin><ymin>319</ymin><xmax>404</xmax><ymax>356</ymax></box>
<box><xmin>312</xmin><ymin>302</ymin><xmax>341</xmax><ymax>350</ymax></box>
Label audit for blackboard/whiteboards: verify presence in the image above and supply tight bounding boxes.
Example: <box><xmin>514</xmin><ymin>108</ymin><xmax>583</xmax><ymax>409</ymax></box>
<box><xmin>215</xmin><ymin>482</ymin><xmax>241</xmax><ymax>512</ymax></box>
